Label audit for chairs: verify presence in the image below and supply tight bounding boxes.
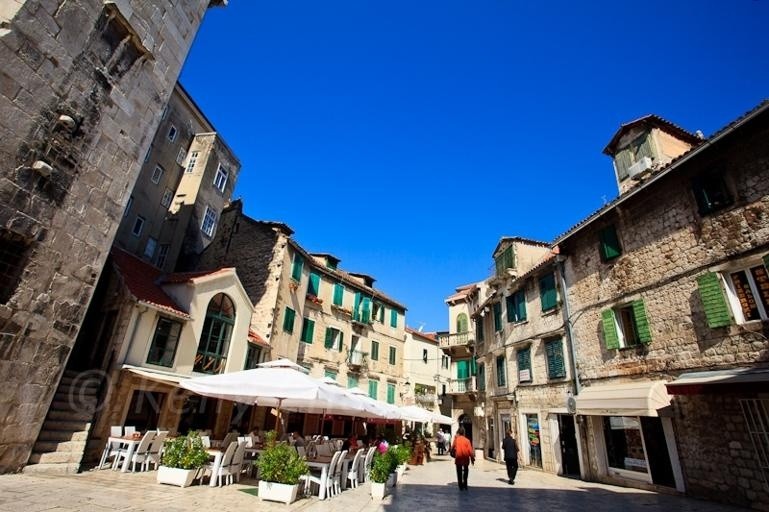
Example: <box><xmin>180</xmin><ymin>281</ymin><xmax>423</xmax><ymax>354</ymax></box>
<box><xmin>99</xmin><ymin>426</ymin><xmax>377</xmax><ymax>500</ymax></box>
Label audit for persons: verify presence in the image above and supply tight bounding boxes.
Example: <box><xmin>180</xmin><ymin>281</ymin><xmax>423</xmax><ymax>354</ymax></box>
<box><xmin>451</xmin><ymin>426</ymin><xmax>474</xmax><ymax>490</ymax></box>
<box><xmin>501</xmin><ymin>429</ymin><xmax>522</xmax><ymax>485</ymax></box>
<box><xmin>436</xmin><ymin>427</ymin><xmax>444</xmax><ymax>455</ymax></box>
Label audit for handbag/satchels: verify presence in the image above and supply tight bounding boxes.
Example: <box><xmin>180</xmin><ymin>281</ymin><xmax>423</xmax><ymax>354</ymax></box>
<box><xmin>451</xmin><ymin>447</ymin><xmax>455</xmax><ymax>458</ymax></box>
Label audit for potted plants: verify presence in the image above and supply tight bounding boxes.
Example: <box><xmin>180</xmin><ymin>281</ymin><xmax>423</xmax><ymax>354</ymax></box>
<box><xmin>370</xmin><ymin>446</ymin><xmax>410</xmax><ymax>498</ymax></box>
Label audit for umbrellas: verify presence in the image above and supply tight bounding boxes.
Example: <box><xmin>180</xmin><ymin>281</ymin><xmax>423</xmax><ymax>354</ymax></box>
<box><xmin>178</xmin><ymin>357</ymin><xmax>457</xmax><ymax>453</ymax></box>
